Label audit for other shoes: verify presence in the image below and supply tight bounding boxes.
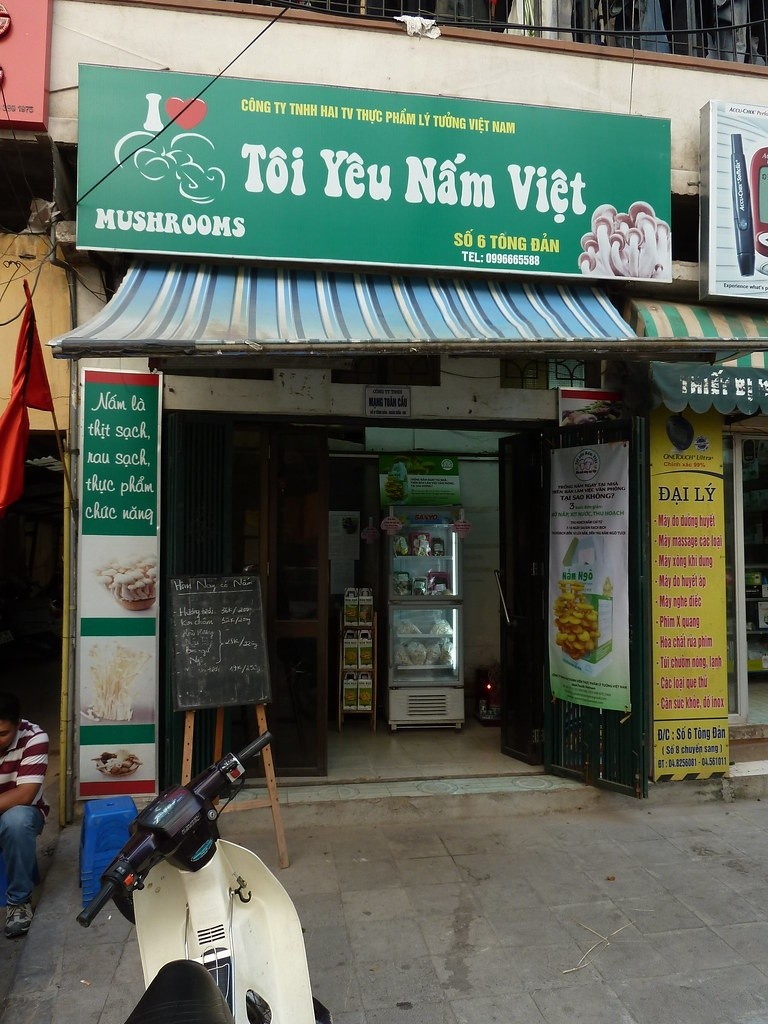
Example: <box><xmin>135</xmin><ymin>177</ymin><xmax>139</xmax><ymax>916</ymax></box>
<box><xmin>4</xmin><ymin>900</ymin><xmax>34</xmax><ymax>938</ymax></box>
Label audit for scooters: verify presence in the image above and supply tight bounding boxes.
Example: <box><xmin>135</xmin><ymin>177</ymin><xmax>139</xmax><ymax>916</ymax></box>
<box><xmin>75</xmin><ymin>729</ymin><xmax>334</xmax><ymax>1024</ymax></box>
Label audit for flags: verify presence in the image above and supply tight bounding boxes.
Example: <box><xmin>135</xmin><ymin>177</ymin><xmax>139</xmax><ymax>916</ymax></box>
<box><xmin>0</xmin><ymin>279</ymin><xmax>53</xmax><ymax>518</ymax></box>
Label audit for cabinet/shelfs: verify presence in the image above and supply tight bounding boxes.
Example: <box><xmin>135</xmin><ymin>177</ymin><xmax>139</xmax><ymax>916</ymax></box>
<box><xmin>337</xmin><ymin>608</ymin><xmax>378</xmax><ymax>735</ymax></box>
<box><xmin>726</xmin><ymin>563</ymin><xmax>768</xmax><ymax>674</ymax></box>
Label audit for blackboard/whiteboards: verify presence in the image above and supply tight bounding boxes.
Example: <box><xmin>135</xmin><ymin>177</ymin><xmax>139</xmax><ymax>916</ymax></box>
<box><xmin>165</xmin><ymin>572</ymin><xmax>274</xmax><ymax>714</ymax></box>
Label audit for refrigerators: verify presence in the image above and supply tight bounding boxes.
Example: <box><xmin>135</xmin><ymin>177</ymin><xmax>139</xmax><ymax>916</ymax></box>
<box><xmin>386</xmin><ymin>503</ymin><xmax>466</xmax><ymax>731</ymax></box>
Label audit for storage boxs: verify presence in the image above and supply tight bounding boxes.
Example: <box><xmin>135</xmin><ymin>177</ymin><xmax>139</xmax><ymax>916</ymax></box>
<box><xmin>342</xmin><ymin>586</ymin><xmax>373</xmax><ymax>711</ymax></box>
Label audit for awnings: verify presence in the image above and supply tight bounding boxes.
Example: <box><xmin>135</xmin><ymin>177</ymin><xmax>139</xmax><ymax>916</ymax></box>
<box><xmin>48</xmin><ymin>264</ymin><xmax>768</xmax><ymax>418</ymax></box>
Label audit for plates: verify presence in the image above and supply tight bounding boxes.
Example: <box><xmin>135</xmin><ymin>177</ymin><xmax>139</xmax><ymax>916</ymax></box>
<box><xmin>95</xmin><ymin>752</ymin><xmax>140</xmax><ymax>779</ymax></box>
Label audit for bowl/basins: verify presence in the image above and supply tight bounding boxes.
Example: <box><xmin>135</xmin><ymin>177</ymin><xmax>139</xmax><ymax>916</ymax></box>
<box><xmin>122</xmin><ymin>597</ymin><xmax>155</xmax><ymax>612</ymax></box>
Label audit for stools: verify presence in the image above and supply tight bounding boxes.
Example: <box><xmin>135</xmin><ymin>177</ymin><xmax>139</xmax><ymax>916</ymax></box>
<box><xmin>77</xmin><ymin>794</ymin><xmax>140</xmax><ymax>909</ymax></box>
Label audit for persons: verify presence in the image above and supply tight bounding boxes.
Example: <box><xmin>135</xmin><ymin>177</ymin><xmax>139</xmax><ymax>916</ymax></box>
<box><xmin>0</xmin><ymin>690</ymin><xmax>50</xmax><ymax>939</ymax></box>
